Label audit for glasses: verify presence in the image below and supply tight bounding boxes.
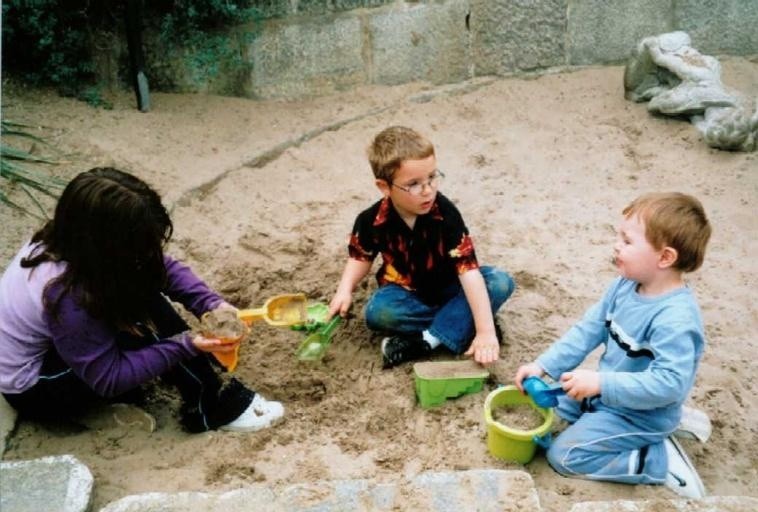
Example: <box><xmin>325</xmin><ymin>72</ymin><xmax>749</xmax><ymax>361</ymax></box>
<box><xmin>390</xmin><ymin>168</ymin><xmax>446</xmax><ymax>196</ymax></box>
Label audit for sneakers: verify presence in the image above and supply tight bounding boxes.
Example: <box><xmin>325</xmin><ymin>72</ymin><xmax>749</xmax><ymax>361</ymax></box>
<box><xmin>217</xmin><ymin>390</ymin><xmax>286</xmax><ymax>434</ymax></box>
<box><xmin>661</xmin><ymin>433</ymin><xmax>707</xmax><ymax>500</ymax></box>
<box><xmin>379</xmin><ymin>329</ymin><xmax>430</xmax><ymax>369</ymax></box>
<box><xmin>672</xmin><ymin>404</ymin><xmax>713</xmax><ymax>445</ymax></box>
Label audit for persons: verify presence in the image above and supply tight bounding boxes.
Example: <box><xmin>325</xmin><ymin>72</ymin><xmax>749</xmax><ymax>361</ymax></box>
<box><xmin>514</xmin><ymin>190</ymin><xmax>713</xmax><ymax>505</ymax></box>
<box><xmin>0</xmin><ymin>167</ymin><xmax>284</xmax><ymax>434</ymax></box>
<box><xmin>324</xmin><ymin>124</ymin><xmax>514</xmax><ymax>371</ymax></box>
